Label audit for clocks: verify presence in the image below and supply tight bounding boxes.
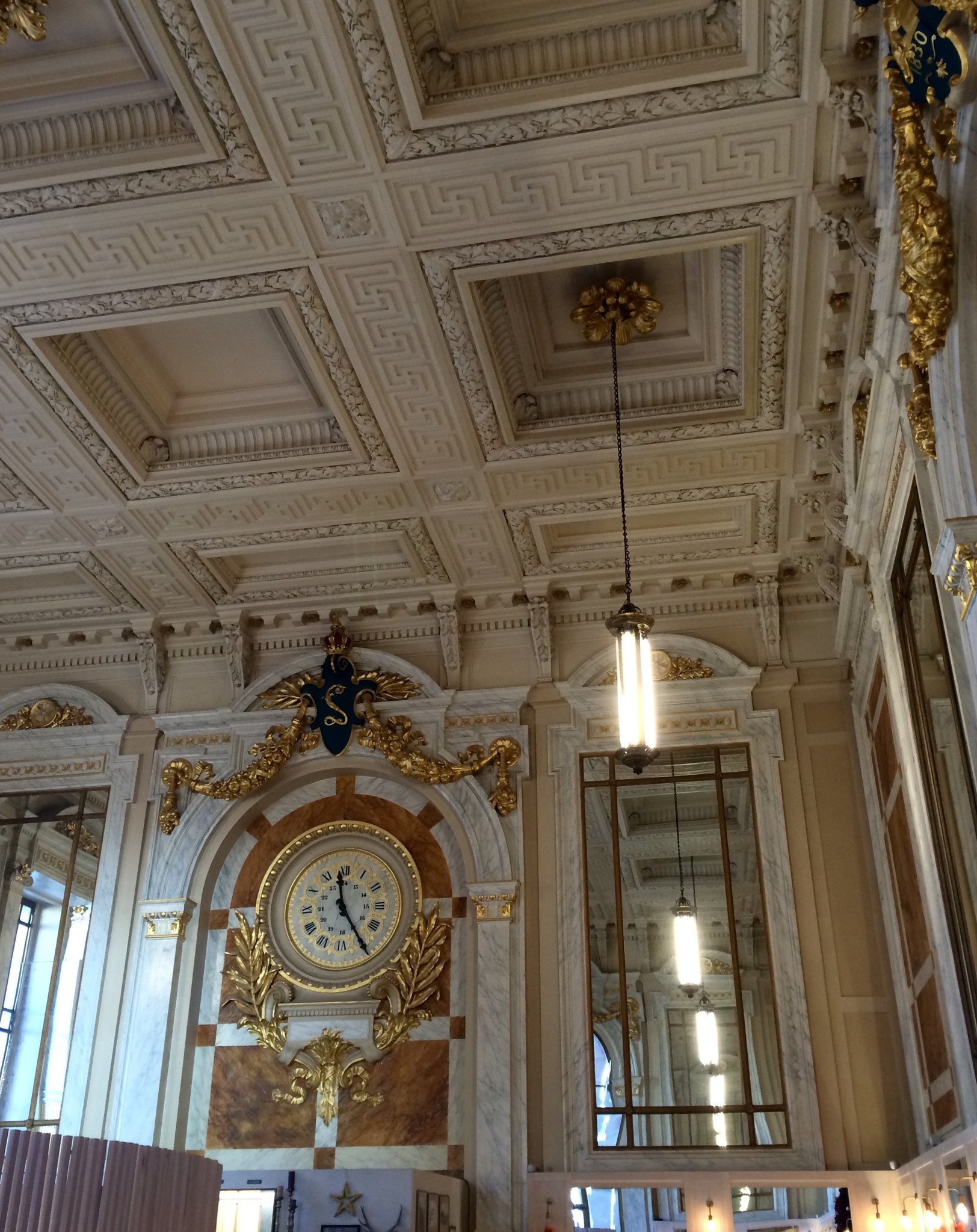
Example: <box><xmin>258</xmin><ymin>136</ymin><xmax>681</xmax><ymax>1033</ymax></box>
<box><xmin>282</xmin><ymin>847</ymin><xmax>403</xmax><ymax>972</ymax></box>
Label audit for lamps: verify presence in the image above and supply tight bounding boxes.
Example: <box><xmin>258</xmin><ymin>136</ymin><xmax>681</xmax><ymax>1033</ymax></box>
<box><xmin>567</xmin><ymin>268</ymin><xmax>977</xmax><ymax>1232</ymax></box>
